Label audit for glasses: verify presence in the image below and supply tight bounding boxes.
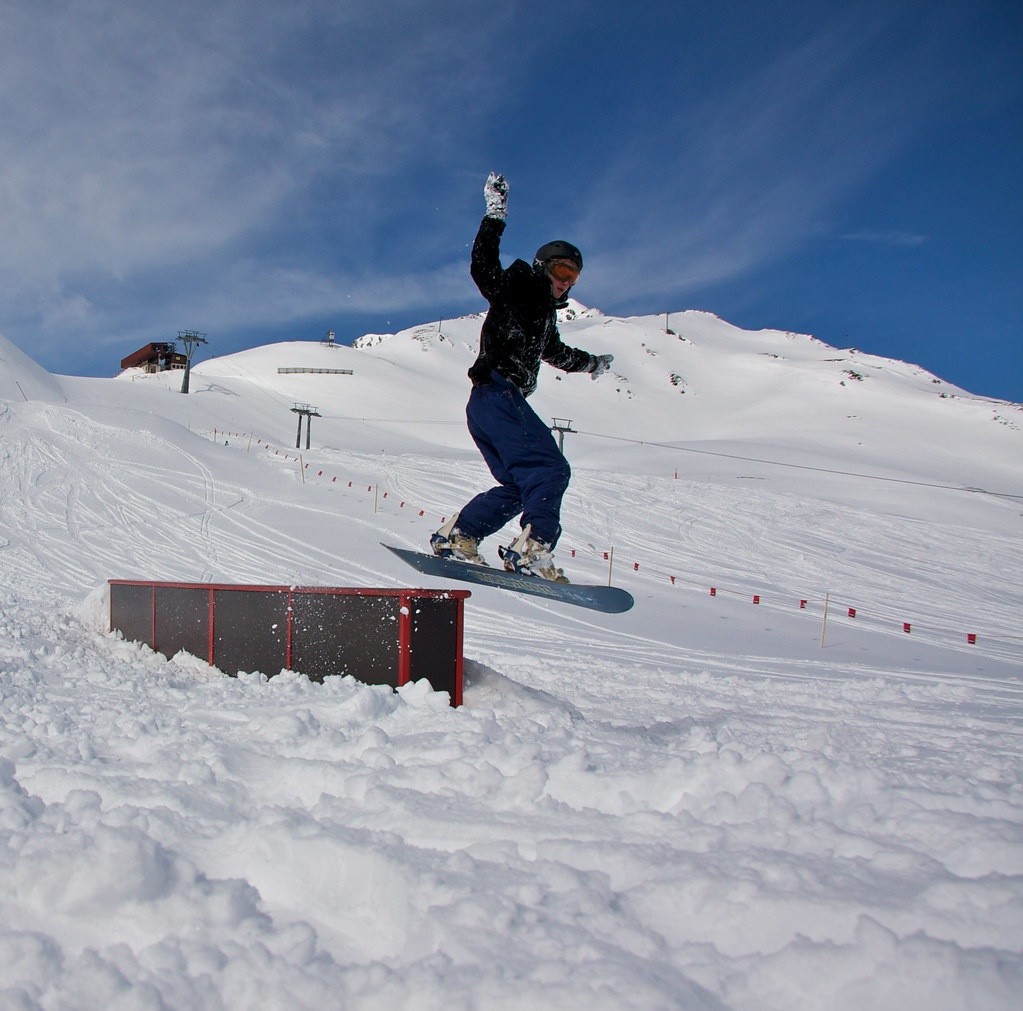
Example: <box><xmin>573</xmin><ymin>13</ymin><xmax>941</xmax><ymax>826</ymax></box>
<box><xmin>546</xmin><ymin>259</ymin><xmax>580</xmax><ymax>286</ymax></box>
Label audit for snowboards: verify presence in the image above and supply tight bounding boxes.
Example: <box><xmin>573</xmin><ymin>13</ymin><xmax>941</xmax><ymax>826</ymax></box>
<box><xmin>379</xmin><ymin>541</ymin><xmax>634</xmax><ymax>614</ymax></box>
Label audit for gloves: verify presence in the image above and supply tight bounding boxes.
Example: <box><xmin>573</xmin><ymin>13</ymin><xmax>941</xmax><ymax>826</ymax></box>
<box><xmin>484</xmin><ymin>172</ymin><xmax>510</xmax><ymax>220</ymax></box>
<box><xmin>584</xmin><ymin>354</ymin><xmax>614</xmax><ymax>380</ymax></box>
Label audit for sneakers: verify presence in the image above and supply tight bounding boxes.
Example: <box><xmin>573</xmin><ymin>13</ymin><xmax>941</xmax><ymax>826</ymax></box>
<box><xmin>437</xmin><ymin>528</ymin><xmax>488</xmax><ymax>567</ymax></box>
<box><xmin>510</xmin><ymin>534</ymin><xmax>568</xmax><ymax>582</ymax></box>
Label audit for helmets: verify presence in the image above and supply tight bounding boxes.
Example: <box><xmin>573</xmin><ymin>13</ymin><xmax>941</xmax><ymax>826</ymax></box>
<box><xmin>533</xmin><ymin>241</ymin><xmax>583</xmax><ymax>276</ymax></box>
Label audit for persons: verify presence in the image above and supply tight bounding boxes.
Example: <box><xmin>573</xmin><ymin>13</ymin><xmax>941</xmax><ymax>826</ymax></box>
<box><xmin>431</xmin><ymin>173</ymin><xmax>613</xmax><ymax>584</ymax></box>
